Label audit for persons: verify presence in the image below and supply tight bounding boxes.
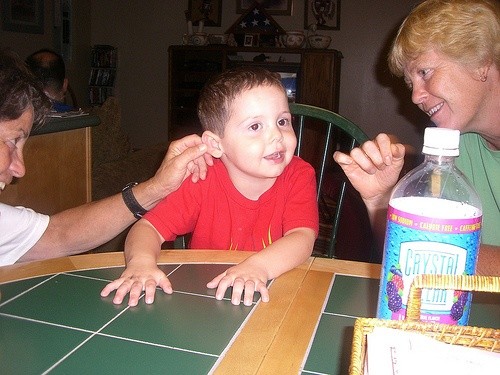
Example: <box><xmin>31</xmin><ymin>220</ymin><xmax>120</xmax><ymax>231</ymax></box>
<box><xmin>0</xmin><ymin>45</ymin><xmax>214</xmax><ymax>269</ymax></box>
<box><xmin>99</xmin><ymin>66</ymin><xmax>319</xmax><ymax>305</ymax></box>
<box><xmin>26</xmin><ymin>50</ymin><xmax>79</xmax><ymax>111</ymax></box>
<box><xmin>331</xmin><ymin>0</ymin><xmax>500</xmax><ymax>275</ymax></box>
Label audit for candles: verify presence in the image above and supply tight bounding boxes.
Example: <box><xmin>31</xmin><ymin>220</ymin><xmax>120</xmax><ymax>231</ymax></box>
<box><xmin>199</xmin><ymin>19</ymin><xmax>204</xmax><ymax>33</ymax></box>
<box><xmin>187</xmin><ymin>19</ymin><xmax>193</xmax><ymax>35</ymax></box>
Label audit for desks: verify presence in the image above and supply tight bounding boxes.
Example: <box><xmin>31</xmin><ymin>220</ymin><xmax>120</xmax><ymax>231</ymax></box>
<box><xmin>0</xmin><ymin>249</ymin><xmax>316</xmax><ymax>375</ymax></box>
<box><xmin>0</xmin><ymin>110</ymin><xmax>100</xmax><ymax>217</ymax></box>
<box><xmin>255</xmin><ymin>257</ymin><xmax>500</xmax><ymax>375</ymax></box>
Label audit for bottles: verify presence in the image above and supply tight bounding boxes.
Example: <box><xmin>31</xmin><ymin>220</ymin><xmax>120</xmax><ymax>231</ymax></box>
<box><xmin>376</xmin><ymin>126</ymin><xmax>484</xmax><ymax>327</ymax></box>
<box><xmin>282</xmin><ymin>30</ymin><xmax>306</xmax><ymax>48</ymax></box>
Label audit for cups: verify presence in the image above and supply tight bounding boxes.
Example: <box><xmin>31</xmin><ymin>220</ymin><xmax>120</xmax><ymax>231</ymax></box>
<box><xmin>193</xmin><ymin>32</ymin><xmax>207</xmax><ymax>45</ymax></box>
<box><xmin>183</xmin><ymin>34</ymin><xmax>192</xmax><ymax>45</ymax></box>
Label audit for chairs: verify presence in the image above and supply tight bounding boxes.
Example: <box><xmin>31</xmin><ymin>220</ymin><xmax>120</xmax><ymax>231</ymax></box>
<box><xmin>173</xmin><ymin>103</ymin><xmax>370</xmax><ymax>264</ymax></box>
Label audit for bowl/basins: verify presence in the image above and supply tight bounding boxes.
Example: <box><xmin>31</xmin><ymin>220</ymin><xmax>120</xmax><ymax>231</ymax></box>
<box><xmin>207</xmin><ymin>34</ymin><xmax>229</xmax><ymax>45</ymax></box>
<box><xmin>307</xmin><ymin>34</ymin><xmax>331</xmax><ymax>49</ymax></box>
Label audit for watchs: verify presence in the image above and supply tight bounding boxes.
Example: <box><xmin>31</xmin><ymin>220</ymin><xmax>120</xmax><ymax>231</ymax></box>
<box><xmin>121</xmin><ymin>181</ymin><xmax>149</xmax><ymax>220</ymax></box>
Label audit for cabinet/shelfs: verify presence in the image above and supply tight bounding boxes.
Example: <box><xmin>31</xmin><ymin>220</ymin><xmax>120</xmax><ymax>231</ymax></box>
<box><xmin>297</xmin><ymin>48</ymin><xmax>345</xmax><ymax>175</ymax></box>
<box><xmin>167</xmin><ymin>44</ymin><xmax>230</xmax><ymax>143</ymax></box>
<box><xmin>87</xmin><ymin>44</ymin><xmax>120</xmax><ymax>107</ymax></box>
<box><xmin>227</xmin><ymin>45</ymin><xmax>302</xmax><ymax>72</ymax></box>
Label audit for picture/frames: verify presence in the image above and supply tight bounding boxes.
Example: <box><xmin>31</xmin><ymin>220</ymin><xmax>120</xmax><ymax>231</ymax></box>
<box><xmin>244</xmin><ymin>34</ymin><xmax>253</xmax><ymax>47</ymax></box>
<box><xmin>0</xmin><ymin>0</ymin><xmax>44</xmax><ymax>35</ymax></box>
<box><xmin>187</xmin><ymin>0</ymin><xmax>222</xmax><ymax>28</ymax></box>
<box><xmin>235</xmin><ymin>0</ymin><xmax>294</xmax><ymax>17</ymax></box>
<box><xmin>303</xmin><ymin>0</ymin><xmax>341</xmax><ymax>31</ymax></box>
